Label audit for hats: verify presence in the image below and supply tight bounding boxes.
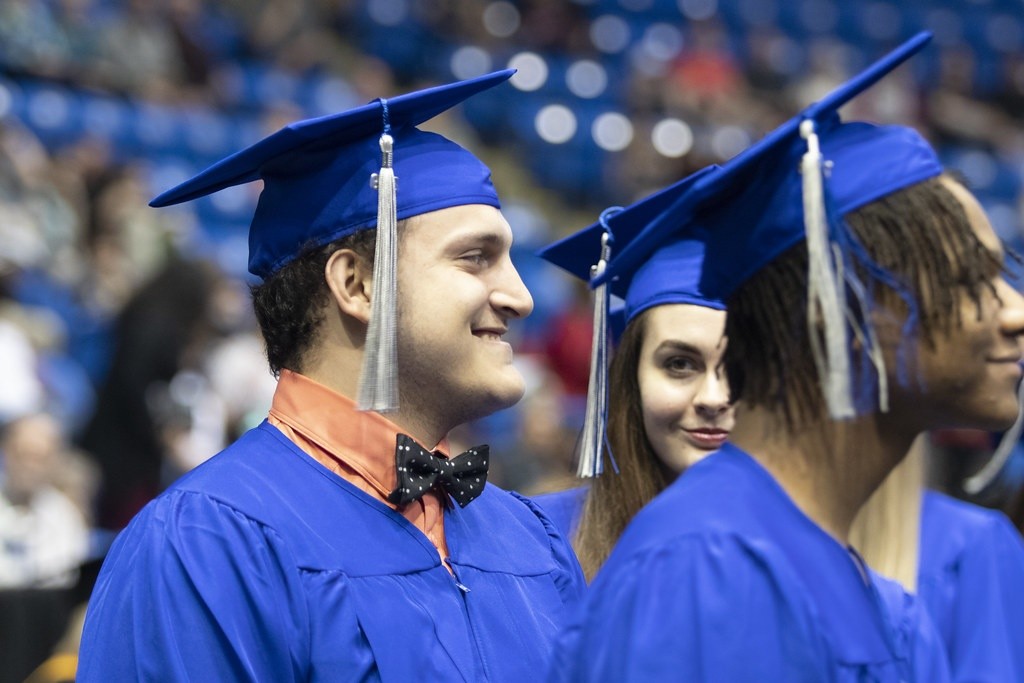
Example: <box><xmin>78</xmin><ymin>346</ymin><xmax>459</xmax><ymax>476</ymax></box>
<box><xmin>539</xmin><ymin>28</ymin><xmax>946</xmax><ymax>321</ymax></box>
<box><xmin>148</xmin><ymin>69</ymin><xmax>518</xmax><ymax>275</ymax></box>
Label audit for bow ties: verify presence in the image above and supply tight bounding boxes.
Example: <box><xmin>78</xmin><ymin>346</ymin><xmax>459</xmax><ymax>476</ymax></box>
<box><xmin>392</xmin><ymin>434</ymin><xmax>494</xmax><ymax>511</ymax></box>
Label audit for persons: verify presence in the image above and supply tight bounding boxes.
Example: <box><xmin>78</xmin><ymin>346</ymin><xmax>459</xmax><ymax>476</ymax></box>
<box><xmin>848</xmin><ymin>423</ymin><xmax>1023</xmax><ymax>683</ymax></box>
<box><xmin>76</xmin><ymin>128</ymin><xmax>589</xmax><ymax>680</ymax></box>
<box><xmin>527</xmin><ymin>236</ymin><xmax>738</xmax><ymax>566</ymax></box>
<box><xmin>278</xmin><ymin>0</ymin><xmax>1024</xmax><ymax>230</ymax></box>
<box><xmin>505</xmin><ymin>381</ymin><xmax>579</xmax><ymax>496</ymax></box>
<box><xmin>1</xmin><ymin>3</ymin><xmax>280</xmax><ymax>614</ymax></box>
<box><xmin>586</xmin><ymin>120</ymin><xmax>1022</xmax><ymax>683</ymax></box>
